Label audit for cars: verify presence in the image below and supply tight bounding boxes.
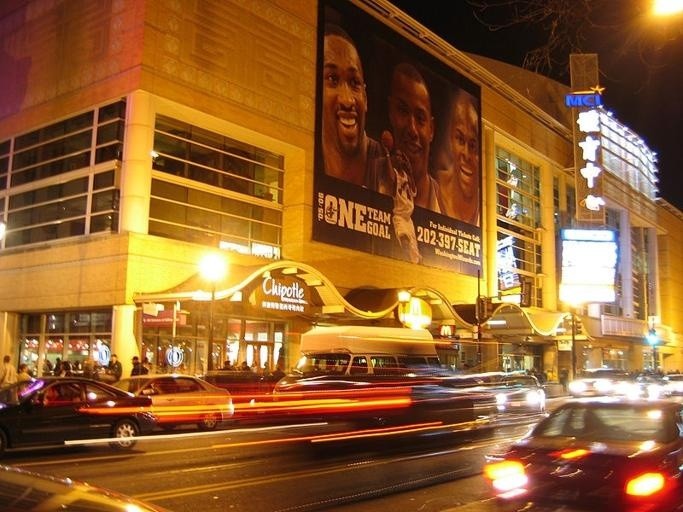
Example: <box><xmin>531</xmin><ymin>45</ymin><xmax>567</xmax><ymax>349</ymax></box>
<box><xmin>108</xmin><ymin>373</ymin><xmax>235</xmax><ymax>433</ymax></box>
<box><xmin>249</xmin><ymin>391</ymin><xmax>272</xmax><ymax>417</ymax></box>
<box><xmin>567</xmin><ymin>367</ymin><xmax>632</xmax><ymax>394</ymax></box>
<box><xmin>625</xmin><ymin>372</ymin><xmax>670</xmax><ymax>397</ymax></box>
<box><xmin>489</xmin><ymin>395</ymin><xmax>683</xmax><ymax>510</ymax></box>
<box><xmin>245</xmin><ymin>375</ymin><xmax>497</xmax><ymax>442</ymax></box>
<box><xmin>663</xmin><ymin>373</ymin><xmax>683</xmax><ymax>395</ymax></box>
<box><xmin>0</xmin><ymin>376</ymin><xmax>155</xmax><ymax>458</ymax></box>
<box><xmin>493</xmin><ymin>374</ymin><xmax>546</xmax><ymax>413</ymax></box>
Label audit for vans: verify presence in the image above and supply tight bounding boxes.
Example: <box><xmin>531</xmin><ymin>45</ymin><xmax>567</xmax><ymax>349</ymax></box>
<box><xmin>272</xmin><ymin>323</ymin><xmax>446</xmax><ymax>424</ymax></box>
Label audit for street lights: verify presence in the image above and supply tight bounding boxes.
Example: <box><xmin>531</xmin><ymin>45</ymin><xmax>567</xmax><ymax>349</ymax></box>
<box><xmin>647</xmin><ymin>328</ymin><xmax>659</xmax><ymax>374</ymax></box>
<box><xmin>192</xmin><ymin>247</ymin><xmax>232</xmax><ymax>377</ymax></box>
<box><xmin>397</xmin><ymin>289</ymin><xmax>412</xmax><ymax>327</ymax></box>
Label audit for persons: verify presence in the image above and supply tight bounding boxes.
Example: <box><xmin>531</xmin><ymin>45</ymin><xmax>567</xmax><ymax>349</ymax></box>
<box><xmin>0</xmin><ymin>354</ymin><xmax>271</xmax><ymax>402</ymax></box>
<box><xmin>324</xmin><ymin>31</ymin><xmax>481</xmax><ymax>263</ymax></box>
<box><xmin>522</xmin><ymin>364</ymin><xmax>681</xmax><ymax>391</ymax></box>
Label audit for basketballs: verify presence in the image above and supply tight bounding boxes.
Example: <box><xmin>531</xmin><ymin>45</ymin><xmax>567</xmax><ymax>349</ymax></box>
<box><xmin>382</xmin><ymin>129</ymin><xmax>392</xmax><ymax>152</ymax></box>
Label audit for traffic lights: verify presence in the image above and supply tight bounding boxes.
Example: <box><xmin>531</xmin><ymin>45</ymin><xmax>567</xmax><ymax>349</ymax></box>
<box><xmin>574</xmin><ymin>318</ymin><xmax>582</xmax><ymax>335</ymax></box>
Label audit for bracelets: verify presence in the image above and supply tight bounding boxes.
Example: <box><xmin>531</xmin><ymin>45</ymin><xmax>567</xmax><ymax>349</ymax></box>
<box><xmin>385</xmin><ymin>153</ymin><xmax>390</xmax><ymax>158</ymax></box>
